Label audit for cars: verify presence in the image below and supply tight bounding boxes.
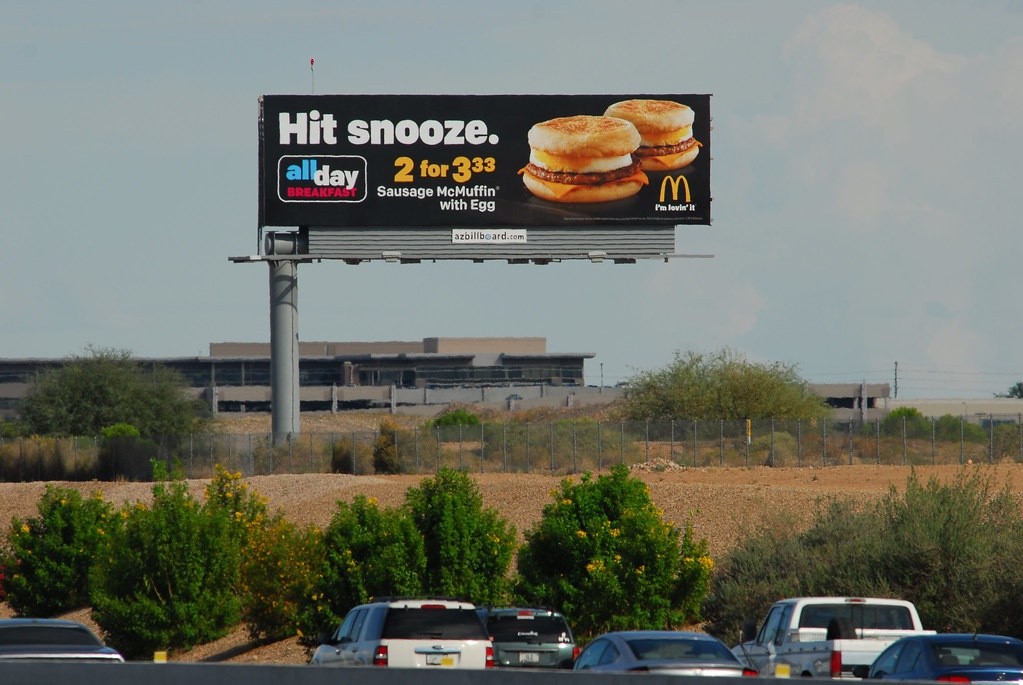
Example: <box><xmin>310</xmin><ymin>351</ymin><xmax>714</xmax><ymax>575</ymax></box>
<box><xmin>852</xmin><ymin>635</ymin><xmax>1023</xmax><ymax>685</ymax></box>
<box><xmin>0</xmin><ymin>618</ymin><xmax>125</xmax><ymax>664</ymax></box>
<box><xmin>572</xmin><ymin>630</ymin><xmax>760</xmax><ymax>679</ymax></box>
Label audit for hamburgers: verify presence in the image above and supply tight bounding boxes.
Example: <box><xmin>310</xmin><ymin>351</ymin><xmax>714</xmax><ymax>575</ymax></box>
<box><xmin>517</xmin><ymin>98</ymin><xmax>701</xmax><ymax>203</ymax></box>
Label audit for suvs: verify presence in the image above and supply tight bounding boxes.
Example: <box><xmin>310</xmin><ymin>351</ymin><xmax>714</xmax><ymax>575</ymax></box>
<box><xmin>476</xmin><ymin>606</ymin><xmax>580</xmax><ymax>669</ymax></box>
<box><xmin>308</xmin><ymin>597</ymin><xmax>495</xmax><ymax>669</ymax></box>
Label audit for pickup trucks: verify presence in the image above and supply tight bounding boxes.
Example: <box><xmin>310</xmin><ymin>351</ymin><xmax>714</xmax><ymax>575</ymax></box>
<box><xmin>730</xmin><ymin>594</ymin><xmax>937</xmax><ymax>681</ymax></box>
